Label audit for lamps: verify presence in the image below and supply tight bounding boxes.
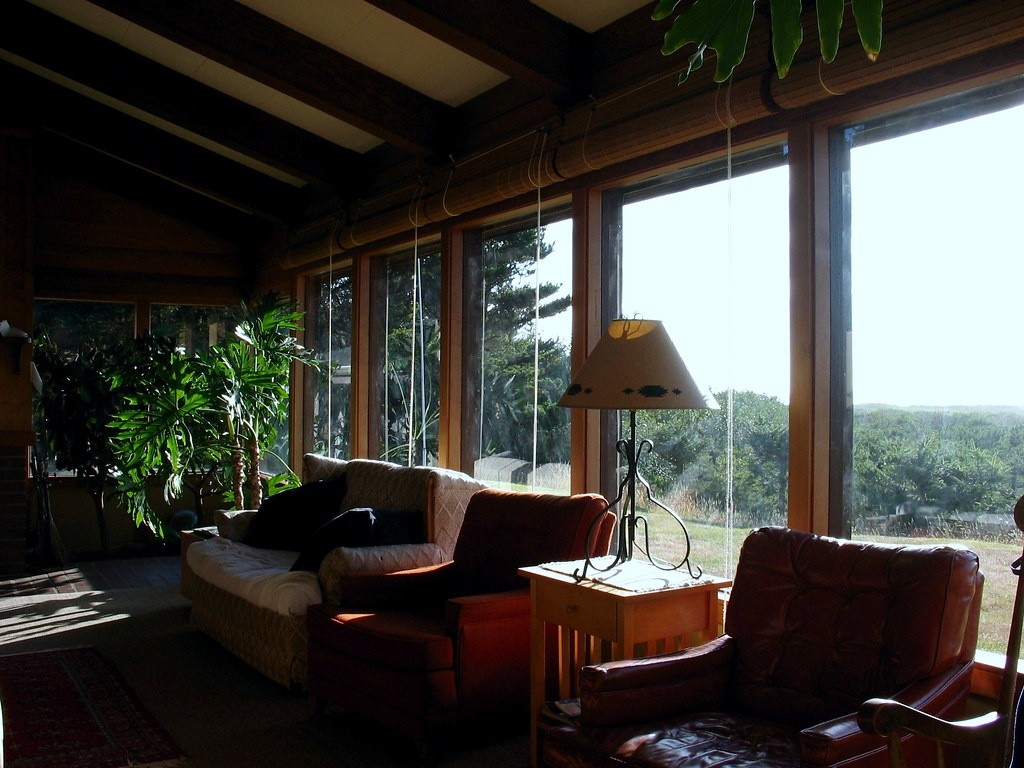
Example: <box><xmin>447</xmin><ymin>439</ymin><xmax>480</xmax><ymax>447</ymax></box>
<box><xmin>555</xmin><ymin>318</ymin><xmax>722</xmax><ymax>583</ymax></box>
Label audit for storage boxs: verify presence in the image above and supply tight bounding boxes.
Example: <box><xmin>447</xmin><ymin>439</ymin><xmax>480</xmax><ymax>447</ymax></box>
<box><xmin>179</xmin><ymin>531</ymin><xmax>209</xmax><ymax>600</ymax></box>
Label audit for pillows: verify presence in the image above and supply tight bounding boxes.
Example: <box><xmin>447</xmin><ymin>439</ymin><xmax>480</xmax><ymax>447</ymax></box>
<box><xmin>238</xmin><ymin>471</ymin><xmax>351</xmax><ymax>549</ymax></box>
<box><xmin>289</xmin><ymin>506</ymin><xmax>427</xmax><ymax>572</ymax></box>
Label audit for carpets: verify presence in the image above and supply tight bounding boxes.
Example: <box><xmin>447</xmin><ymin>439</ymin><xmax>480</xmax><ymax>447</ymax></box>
<box><xmin>0</xmin><ymin>642</ymin><xmax>193</xmax><ymax>768</ymax></box>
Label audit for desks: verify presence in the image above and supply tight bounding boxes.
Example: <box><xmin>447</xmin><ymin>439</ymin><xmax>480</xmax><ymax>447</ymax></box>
<box><xmin>516</xmin><ymin>555</ymin><xmax>733</xmax><ymax>768</ymax></box>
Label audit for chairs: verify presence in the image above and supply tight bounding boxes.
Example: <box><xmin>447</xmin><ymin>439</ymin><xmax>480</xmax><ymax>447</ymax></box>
<box><xmin>854</xmin><ymin>496</ymin><xmax>1024</xmax><ymax>768</ymax></box>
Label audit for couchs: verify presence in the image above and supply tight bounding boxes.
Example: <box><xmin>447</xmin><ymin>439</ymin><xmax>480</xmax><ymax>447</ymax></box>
<box><xmin>191</xmin><ymin>453</ymin><xmax>439</xmax><ymax>693</ymax></box>
<box><xmin>305</xmin><ymin>491</ymin><xmax>615</xmax><ymax>768</ymax></box>
<box><xmin>578</xmin><ymin>527</ymin><xmax>987</xmax><ymax>768</ymax></box>
